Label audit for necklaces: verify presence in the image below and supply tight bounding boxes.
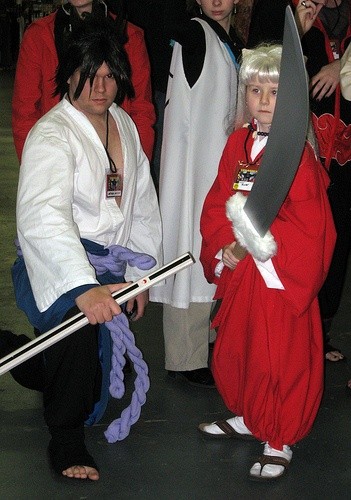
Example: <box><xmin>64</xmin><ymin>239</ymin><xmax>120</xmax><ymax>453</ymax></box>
<box><xmin>256</xmin><ymin>131</ymin><xmax>269</xmax><ymax>137</ymax></box>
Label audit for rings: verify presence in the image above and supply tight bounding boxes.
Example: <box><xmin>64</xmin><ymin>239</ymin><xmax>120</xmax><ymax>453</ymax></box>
<box><xmin>301</xmin><ymin>1</ymin><xmax>306</xmax><ymax>7</ymax></box>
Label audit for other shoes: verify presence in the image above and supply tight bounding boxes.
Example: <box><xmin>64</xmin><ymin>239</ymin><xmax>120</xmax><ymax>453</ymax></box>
<box><xmin>182</xmin><ymin>366</ymin><xmax>217</xmax><ymax>388</ymax></box>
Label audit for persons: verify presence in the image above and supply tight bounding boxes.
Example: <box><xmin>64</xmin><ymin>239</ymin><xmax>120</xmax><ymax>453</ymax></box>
<box><xmin>12</xmin><ymin>0</ymin><xmax>155</xmax><ymax>163</ymax></box>
<box><xmin>249</xmin><ymin>0</ymin><xmax>351</xmax><ymax>363</ymax></box>
<box><xmin>198</xmin><ymin>45</ymin><xmax>336</xmax><ymax>481</ymax></box>
<box><xmin>340</xmin><ymin>42</ymin><xmax>351</xmax><ymax>391</ymax></box>
<box><xmin>149</xmin><ymin>0</ymin><xmax>252</xmax><ymax>387</ymax></box>
<box><xmin>0</xmin><ymin>14</ymin><xmax>162</xmax><ymax>482</ymax></box>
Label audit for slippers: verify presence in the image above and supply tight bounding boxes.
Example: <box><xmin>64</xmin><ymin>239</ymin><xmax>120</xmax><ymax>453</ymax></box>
<box><xmin>249</xmin><ymin>453</ymin><xmax>288</xmax><ymax>482</ymax></box>
<box><xmin>198</xmin><ymin>420</ymin><xmax>256</xmax><ymax>440</ymax></box>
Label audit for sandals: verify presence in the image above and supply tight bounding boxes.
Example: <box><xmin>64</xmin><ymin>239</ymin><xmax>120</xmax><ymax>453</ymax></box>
<box><xmin>324</xmin><ymin>342</ymin><xmax>344</xmax><ymax>363</ymax></box>
<box><xmin>47</xmin><ymin>438</ymin><xmax>101</xmax><ymax>483</ymax></box>
